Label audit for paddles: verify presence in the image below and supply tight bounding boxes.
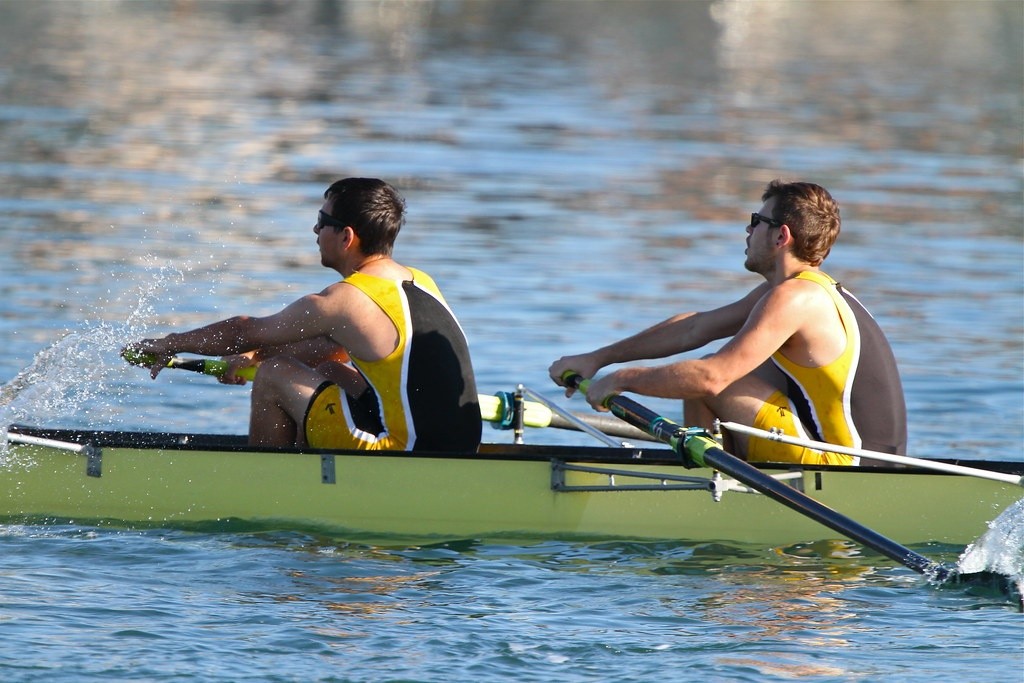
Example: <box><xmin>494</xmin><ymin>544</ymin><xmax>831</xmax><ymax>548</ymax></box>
<box><xmin>122</xmin><ymin>347</ymin><xmax>670</xmax><ymax>444</ymax></box>
<box><xmin>558</xmin><ymin>367</ymin><xmax>1024</xmax><ymax>612</ymax></box>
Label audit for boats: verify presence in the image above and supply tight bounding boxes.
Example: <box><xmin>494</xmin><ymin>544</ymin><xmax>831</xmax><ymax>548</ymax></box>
<box><xmin>0</xmin><ymin>421</ymin><xmax>1024</xmax><ymax>556</ymax></box>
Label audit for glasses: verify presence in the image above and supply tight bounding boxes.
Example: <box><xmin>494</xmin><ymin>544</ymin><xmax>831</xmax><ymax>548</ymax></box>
<box><xmin>750</xmin><ymin>213</ymin><xmax>798</xmax><ymax>239</ymax></box>
<box><xmin>317</xmin><ymin>210</ymin><xmax>346</xmax><ymax>229</ymax></box>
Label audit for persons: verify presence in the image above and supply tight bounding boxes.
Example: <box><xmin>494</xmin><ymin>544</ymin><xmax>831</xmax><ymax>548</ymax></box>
<box><xmin>548</xmin><ymin>181</ymin><xmax>909</xmax><ymax>468</ymax></box>
<box><xmin>119</xmin><ymin>177</ymin><xmax>483</xmax><ymax>454</ymax></box>
